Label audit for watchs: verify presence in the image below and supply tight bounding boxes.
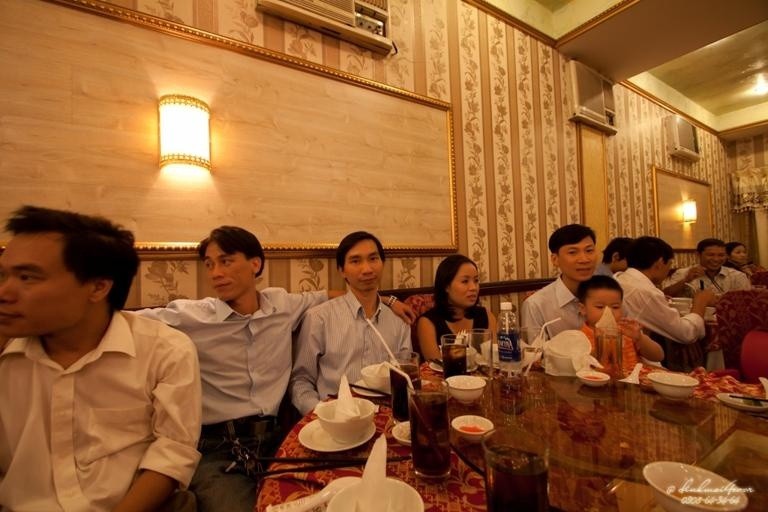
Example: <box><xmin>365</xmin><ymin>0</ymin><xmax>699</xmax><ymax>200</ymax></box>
<box><xmin>386</xmin><ymin>295</ymin><xmax>397</xmax><ymax>307</ymax></box>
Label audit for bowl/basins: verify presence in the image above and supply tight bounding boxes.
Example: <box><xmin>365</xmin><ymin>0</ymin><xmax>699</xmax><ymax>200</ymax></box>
<box><xmin>672</xmin><ymin>297</ymin><xmax>692</xmax><ymax>305</ymax></box>
<box><xmin>317</xmin><ymin>398</ymin><xmax>376</xmax><ymax>442</ymax></box>
<box><xmin>647</xmin><ymin>373</ymin><xmax>700</xmax><ymax>401</ymax></box>
<box><xmin>669</xmin><ymin>302</ymin><xmax>687</xmax><ymax>310</ymax></box>
<box><xmin>642</xmin><ymin>460</ymin><xmax>748</xmax><ymax>512</ymax></box>
<box><xmin>360</xmin><ymin>363</ymin><xmax>391</xmax><ymax>390</ymax></box>
<box><xmin>446</xmin><ymin>375</ymin><xmax>487</xmax><ymax>403</ymax></box>
<box><xmin>577</xmin><ymin>371</ymin><xmax>610</xmax><ymax>388</ymax></box>
<box><xmin>451</xmin><ymin>415</ymin><xmax>494</xmax><ymax>440</ymax></box>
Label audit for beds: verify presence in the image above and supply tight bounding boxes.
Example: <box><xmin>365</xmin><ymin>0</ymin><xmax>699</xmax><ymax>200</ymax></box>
<box><xmin>253</xmin><ymin>359</ymin><xmax>768</xmax><ymax>512</ymax></box>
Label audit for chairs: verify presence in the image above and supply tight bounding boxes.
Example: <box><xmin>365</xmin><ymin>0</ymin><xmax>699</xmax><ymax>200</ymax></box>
<box><xmin>406</xmin><ymin>294</ymin><xmax>441</xmax><ymax>352</ymax></box>
<box><xmin>741</xmin><ymin>263</ymin><xmax>768</xmax><ymax>286</ymax></box>
<box><xmin>704</xmin><ymin>288</ymin><xmax>768</xmax><ymax>373</ymax></box>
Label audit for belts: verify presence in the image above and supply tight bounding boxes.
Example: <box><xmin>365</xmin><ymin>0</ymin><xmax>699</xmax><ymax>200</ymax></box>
<box><xmin>200</xmin><ymin>419</ymin><xmax>281</xmax><ymax>438</ymax></box>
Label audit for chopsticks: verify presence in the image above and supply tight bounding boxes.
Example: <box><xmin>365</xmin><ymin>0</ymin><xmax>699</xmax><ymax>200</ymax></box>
<box><xmin>704</xmin><ymin>270</ymin><xmax>724</xmax><ymax>294</ymax></box>
<box><xmin>255</xmin><ymin>456</ymin><xmax>413</xmax><ymax>476</ymax></box>
<box><xmin>729</xmin><ymin>394</ymin><xmax>768</xmax><ymax>401</ymax></box>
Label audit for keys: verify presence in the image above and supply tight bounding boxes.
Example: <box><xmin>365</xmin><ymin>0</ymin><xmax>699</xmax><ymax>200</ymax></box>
<box><xmin>222</xmin><ymin>436</ymin><xmax>260</xmax><ymax>475</ymax></box>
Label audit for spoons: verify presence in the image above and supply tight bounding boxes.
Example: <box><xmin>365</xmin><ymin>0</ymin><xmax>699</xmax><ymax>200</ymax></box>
<box><xmin>267</xmin><ymin>477</ymin><xmax>361</xmax><ymax>512</ymax></box>
<box><xmin>619</xmin><ymin>363</ymin><xmax>645</xmax><ymax>391</ymax></box>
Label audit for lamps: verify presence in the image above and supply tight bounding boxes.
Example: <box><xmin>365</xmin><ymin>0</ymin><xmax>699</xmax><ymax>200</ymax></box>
<box><xmin>681</xmin><ymin>197</ymin><xmax>700</xmax><ymax>224</ymax></box>
<box><xmin>158</xmin><ymin>93</ymin><xmax>211</xmax><ymax>172</ymax></box>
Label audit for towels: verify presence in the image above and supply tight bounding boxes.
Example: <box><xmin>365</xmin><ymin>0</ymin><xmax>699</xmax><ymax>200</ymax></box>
<box><xmin>357</xmin><ymin>433</ymin><xmax>388</xmax><ymax>512</ymax></box>
<box><xmin>375</xmin><ymin>362</ymin><xmax>391</xmax><ymax>376</ymax></box>
<box><xmin>455</xmin><ymin>331</ymin><xmax>471</xmax><ymax>349</ymax></box>
<box><xmin>334</xmin><ymin>373</ymin><xmax>362</xmax><ymax>423</ymax></box>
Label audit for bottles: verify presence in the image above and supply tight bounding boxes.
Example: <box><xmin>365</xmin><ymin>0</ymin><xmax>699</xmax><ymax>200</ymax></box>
<box><xmin>497</xmin><ymin>302</ymin><xmax>522</xmax><ymax>376</ymax></box>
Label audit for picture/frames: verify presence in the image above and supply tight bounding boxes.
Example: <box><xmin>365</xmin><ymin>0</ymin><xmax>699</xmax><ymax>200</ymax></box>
<box><xmin>651</xmin><ymin>166</ymin><xmax>714</xmax><ymax>251</ymax></box>
<box><xmin>2</xmin><ymin>2</ymin><xmax>471</xmax><ymax>261</ymax></box>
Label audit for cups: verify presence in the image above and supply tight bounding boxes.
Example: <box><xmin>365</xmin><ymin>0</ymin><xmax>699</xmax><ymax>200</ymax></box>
<box><xmin>442</xmin><ymin>334</ymin><xmax>467</xmax><ymax>376</ymax></box>
<box><xmin>521</xmin><ymin>327</ymin><xmax>545</xmax><ymax>371</ymax></box>
<box><xmin>390</xmin><ymin>351</ymin><xmax>419</xmax><ymax>421</ymax></box>
<box><xmin>408</xmin><ymin>375</ymin><xmax>452</xmax><ymax>479</ymax></box>
<box><xmin>481</xmin><ymin>426</ymin><xmax>549</xmax><ymax>512</ymax></box>
<box><xmin>595</xmin><ymin>327</ymin><xmax>623</xmax><ymax>384</ymax></box>
<box><xmin>466</xmin><ymin>328</ymin><xmax>494</xmax><ymax>375</ymax></box>
<box><xmin>492</xmin><ymin>374</ymin><xmax>530</xmax><ymax>415</ymax></box>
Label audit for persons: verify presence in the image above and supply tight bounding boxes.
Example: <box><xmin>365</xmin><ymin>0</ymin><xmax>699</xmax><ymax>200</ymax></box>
<box><xmin>0</xmin><ymin>204</ymin><xmax>202</xmax><ymax>512</ymax></box>
<box><xmin>725</xmin><ymin>241</ymin><xmax>750</xmax><ymax>274</ymax></box>
<box><xmin>612</xmin><ymin>236</ymin><xmax>716</xmax><ymax>344</ymax></box>
<box><xmin>122</xmin><ymin>225</ymin><xmax>416</xmax><ymax>512</ymax></box>
<box><xmin>593</xmin><ymin>237</ymin><xmax>636</xmax><ymax>276</ymax></box>
<box><xmin>661</xmin><ymin>238</ymin><xmax>752</xmax><ymax>297</ymax></box>
<box><xmin>521</xmin><ymin>224</ymin><xmax>623</xmax><ymax>345</ymax></box>
<box><xmin>291</xmin><ymin>231</ymin><xmax>413</xmax><ymax>417</ymax></box>
<box><xmin>416</xmin><ymin>254</ymin><xmax>497</xmax><ymax>361</ymax></box>
<box><xmin>577</xmin><ymin>275</ymin><xmax>664</xmax><ymax>370</ymax></box>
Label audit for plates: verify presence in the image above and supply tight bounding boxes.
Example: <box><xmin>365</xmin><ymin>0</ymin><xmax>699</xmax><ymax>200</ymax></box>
<box><xmin>298</xmin><ymin>419</ymin><xmax>377</xmax><ymax>453</ymax></box>
<box><xmin>716</xmin><ymin>392</ymin><xmax>768</xmax><ymax>412</ymax></box>
<box><xmin>327</xmin><ymin>477</ymin><xmax>424</xmax><ymax>512</ymax></box>
<box><xmin>430</xmin><ymin>358</ymin><xmax>481</xmax><ymax>374</ymax></box>
<box><xmin>352</xmin><ymin>380</ymin><xmax>390</xmax><ymax>397</ymax></box>
<box><xmin>392</xmin><ymin>421</ymin><xmax>412</xmax><ymax>446</ymax></box>
